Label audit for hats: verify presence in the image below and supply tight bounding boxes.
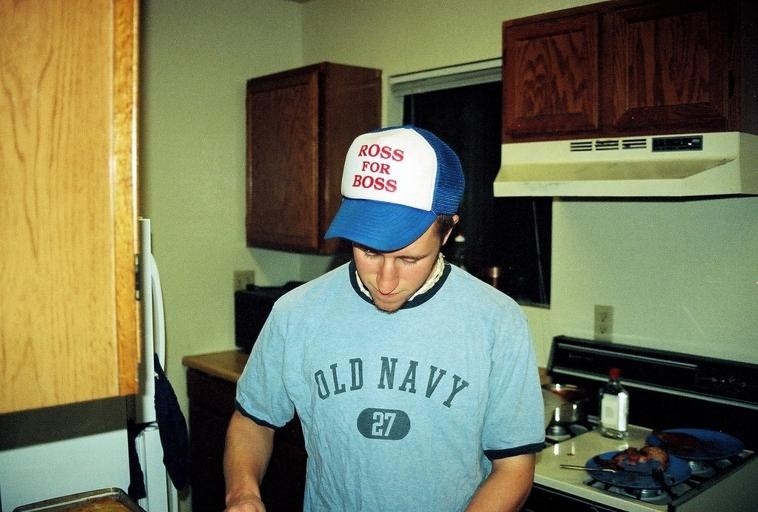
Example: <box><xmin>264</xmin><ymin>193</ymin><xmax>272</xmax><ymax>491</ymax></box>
<box><xmin>322</xmin><ymin>125</ymin><xmax>467</xmax><ymax>253</ymax></box>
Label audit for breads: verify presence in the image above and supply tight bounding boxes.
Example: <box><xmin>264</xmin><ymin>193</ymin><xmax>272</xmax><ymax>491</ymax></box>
<box><xmin>59</xmin><ymin>497</ymin><xmax>134</xmax><ymax>512</ymax></box>
<box><xmin>612</xmin><ymin>446</ymin><xmax>669</xmax><ymax>466</ymax></box>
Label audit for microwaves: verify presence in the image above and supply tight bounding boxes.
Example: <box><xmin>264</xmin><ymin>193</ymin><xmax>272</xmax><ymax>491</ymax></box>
<box><xmin>231</xmin><ymin>279</ymin><xmax>313</xmax><ymax>353</ymax></box>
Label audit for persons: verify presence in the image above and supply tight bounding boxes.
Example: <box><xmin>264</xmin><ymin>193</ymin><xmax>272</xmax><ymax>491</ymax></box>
<box><xmin>222</xmin><ymin>125</ymin><xmax>543</xmax><ymax>511</ymax></box>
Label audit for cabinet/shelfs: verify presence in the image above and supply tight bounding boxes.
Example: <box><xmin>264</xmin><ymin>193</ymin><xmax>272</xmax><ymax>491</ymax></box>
<box><xmin>244</xmin><ymin>61</ymin><xmax>383</xmax><ymax>255</ymax></box>
<box><xmin>502</xmin><ymin>0</ymin><xmax>758</xmax><ymax>144</ymax></box>
<box><xmin>181</xmin><ymin>369</ymin><xmax>305</xmax><ymax>512</ymax></box>
<box><xmin>2</xmin><ymin>4</ymin><xmax>142</xmax><ymax>418</ymax></box>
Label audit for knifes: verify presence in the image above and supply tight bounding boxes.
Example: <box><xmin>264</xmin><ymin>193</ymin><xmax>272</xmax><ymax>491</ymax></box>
<box><xmin>559</xmin><ymin>463</ymin><xmax>651</xmax><ymax>478</ymax></box>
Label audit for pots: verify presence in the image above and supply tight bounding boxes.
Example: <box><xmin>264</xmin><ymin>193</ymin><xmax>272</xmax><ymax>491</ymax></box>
<box><xmin>541</xmin><ymin>382</ymin><xmax>590</xmax><ymax>425</ymax></box>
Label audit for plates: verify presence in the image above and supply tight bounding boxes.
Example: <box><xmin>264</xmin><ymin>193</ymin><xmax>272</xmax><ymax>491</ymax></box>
<box><xmin>583</xmin><ymin>448</ymin><xmax>693</xmax><ymax>491</ymax></box>
<box><xmin>645</xmin><ymin>427</ymin><xmax>744</xmax><ymax>461</ymax></box>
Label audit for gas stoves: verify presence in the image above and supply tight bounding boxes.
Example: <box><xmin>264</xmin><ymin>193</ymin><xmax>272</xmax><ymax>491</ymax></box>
<box><xmin>530</xmin><ymin>411</ymin><xmax>758</xmax><ymax>512</ymax></box>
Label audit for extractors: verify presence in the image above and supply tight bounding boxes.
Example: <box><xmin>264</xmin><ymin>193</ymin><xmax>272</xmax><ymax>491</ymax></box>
<box><xmin>493</xmin><ymin>131</ymin><xmax>758</xmax><ymax>203</ymax></box>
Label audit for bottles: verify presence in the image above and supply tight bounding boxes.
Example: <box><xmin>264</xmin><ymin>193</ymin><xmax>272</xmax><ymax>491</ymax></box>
<box><xmin>597</xmin><ymin>367</ymin><xmax>630</xmax><ymax>440</ymax></box>
